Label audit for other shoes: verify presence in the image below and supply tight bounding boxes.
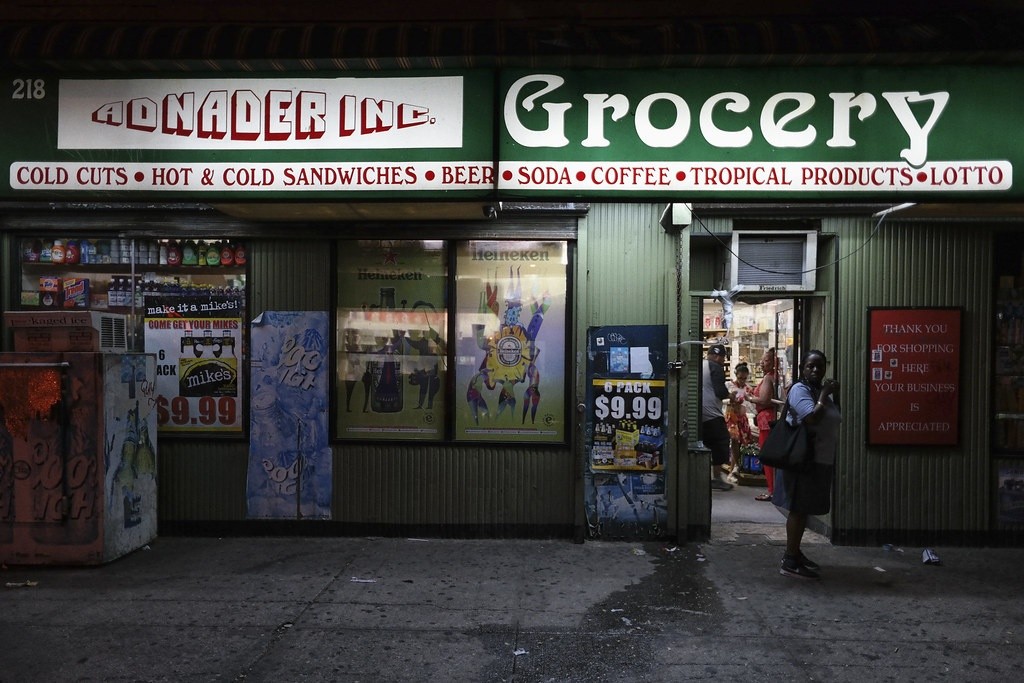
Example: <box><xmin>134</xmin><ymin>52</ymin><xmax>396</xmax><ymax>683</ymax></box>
<box><xmin>727</xmin><ymin>466</ymin><xmax>739</xmax><ymax>483</ymax></box>
<box><xmin>711</xmin><ymin>478</ymin><xmax>733</xmax><ymax>490</ymax></box>
<box><xmin>755</xmin><ymin>493</ymin><xmax>773</xmax><ymax>501</ymax></box>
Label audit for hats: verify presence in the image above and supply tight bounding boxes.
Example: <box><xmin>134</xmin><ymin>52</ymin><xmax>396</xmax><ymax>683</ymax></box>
<box><xmin>708</xmin><ymin>345</ymin><xmax>727</xmax><ymax>356</ymax></box>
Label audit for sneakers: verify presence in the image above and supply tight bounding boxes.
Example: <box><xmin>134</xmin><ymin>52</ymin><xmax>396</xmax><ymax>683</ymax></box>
<box><xmin>779</xmin><ymin>550</ymin><xmax>820</xmax><ymax>580</ymax></box>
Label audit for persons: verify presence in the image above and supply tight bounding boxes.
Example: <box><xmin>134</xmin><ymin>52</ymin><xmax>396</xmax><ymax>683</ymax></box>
<box><xmin>779</xmin><ymin>350</ymin><xmax>840</xmax><ymax>581</ymax></box>
<box><xmin>744</xmin><ymin>347</ymin><xmax>779</xmax><ymax>501</ymax></box>
<box><xmin>722</xmin><ymin>362</ymin><xmax>755</xmax><ymax>483</ymax></box>
<box><xmin>701</xmin><ymin>345</ymin><xmax>733</xmax><ymax>491</ymax></box>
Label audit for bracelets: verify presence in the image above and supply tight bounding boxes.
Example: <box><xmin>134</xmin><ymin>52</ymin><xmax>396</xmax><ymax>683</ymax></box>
<box><xmin>817</xmin><ymin>401</ymin><xmax>825</xmax><ymax>408</ymax></box>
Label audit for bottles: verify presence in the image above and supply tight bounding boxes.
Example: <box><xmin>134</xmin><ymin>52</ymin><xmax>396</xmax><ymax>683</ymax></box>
<box><xmin>370</xmin><ymin>287</ymin><xmax>403</xmax><ymax>412</ymax></box>
<box><xmin>52</xmin><ymin>241</ymin><xmax>65</xmax><ymax>263</ymax></box>
<box><xmin>253</xmin><ymin>391</ymin><xmax>314</xmax><ymax>503</ymax></box>
<box><xmin>79</xmin><ymin>240</ymin><xmax>89</xmax><ymax>263</ymax></box>
<box><xmin>65</xmin><ymin>241</ymin><xmax>80</xmax><ymax>263</ymax></box>
<box><xmin>200</xmin><ymin>330</ymin><xmax>217</xmax><ymax>358</ymax></box>
<box><xmin>107</xmin><ymin>279</ymin><xmax>132</xmax><ymax>307</ymax></box>
<box><xmin>110</xmin><ymin>414</ymin><xmax>138</xmax><ymax>512</ymax></box>
<box><xmin>0</xmin><ymin>405</ymin><xmax>15</xmax><ymax>544</ymax></box>
<box><xmin>218</xmin><ymin>330</ymin><xmax>237</xmax><ymax>358</ymax></box>
<box><xmin>179</xmin><ymin>330</ymin><xmax>197</xmax><ymax>358</ymax></box>
<box><xmin>30</xmin><ymin>347</ymin><xmax>100</xmax><ymax>544</ymax></box>
<box><xmin>737</xmin><ymin>444</ymin><xmax>762</xmax><ymax>476</ymax></box>
<box><xmin>136</xmin><ymin>416</ymin><xmax>156</xmax><ymax>478</ymax></box>
<box><xmin>135</xmin><ymin>274</ymin><xmax>245</xmax><ymax>318</ymax></box>
<box><xmin>591</xmin><ymin>419</ymin><xmax>661</xmax><ymax>466</ymax></box>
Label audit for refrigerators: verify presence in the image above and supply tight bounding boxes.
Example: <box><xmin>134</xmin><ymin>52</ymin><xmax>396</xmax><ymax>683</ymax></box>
<box><xmin>0</xmin><ymin>352</ymin><xmax>158</xmax><ymax>565</ymax></box>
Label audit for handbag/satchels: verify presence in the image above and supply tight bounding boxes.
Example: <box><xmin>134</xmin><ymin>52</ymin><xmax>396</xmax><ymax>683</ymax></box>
<box><xmin>757</xmin><ymin>382</ymin><xmax>814</xmax><ymax>472</ymax></box>
